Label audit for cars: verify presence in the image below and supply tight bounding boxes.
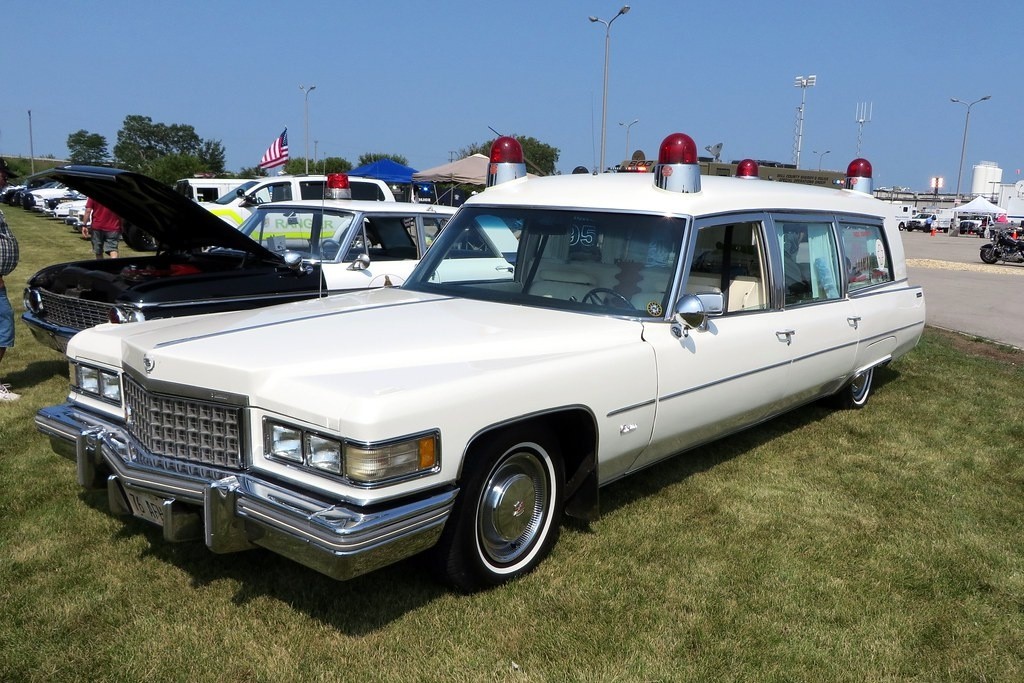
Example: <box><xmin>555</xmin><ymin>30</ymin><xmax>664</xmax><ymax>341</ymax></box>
<box><xmin>20</xmin><ymin>163</ymin><xmax>524</xmax><ymax>353</ymax></box>
<box><xmin>977</xmin><ymin>222</ymin><xmax>1023</xmax><ymax>239</ymax></box>
<box><xmin>3</xmin><ymin>182</ymin><xmax>92</xmax><ymax>234</ymax></box>
<box><xmin>34</xmin><ymin>132</ymin><xmax>925</xmax><ymax>584</ymax></box>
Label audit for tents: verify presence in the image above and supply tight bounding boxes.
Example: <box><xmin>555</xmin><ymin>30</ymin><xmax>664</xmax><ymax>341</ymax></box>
<box><xmin>948</xmin><ymin>195</ymin><xmax>1008</xmax><ymax>238</ymax></box>
<box><xmin>342</xmin><ymin>157</ymin><xmax>439</xmax><ymax>205</ymax></box>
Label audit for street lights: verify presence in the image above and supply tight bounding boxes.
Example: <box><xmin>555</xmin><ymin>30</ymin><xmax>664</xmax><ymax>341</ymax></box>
<box><xmin>950</xmin><ymin>96</ymin><xmax>991</xmax><ymax>229</ymax></box>
<box><xmin>618</xmin><ymin>119</ymin><xmax>638</xmax><ymax>162</ymax></box>
<box><xmin>589</xmin><ymin>6</ymin><xmax>631</xmax><ymax>173</ymax></box>
<box><xmin>812</xmin><ymin>151</ymin><xmax>830</xmax><ymax>171</ymax></box>
<box><xmin>298</xmin><ymin>84</ymin><xmax>315</xmax><ymax>174</ymax></box>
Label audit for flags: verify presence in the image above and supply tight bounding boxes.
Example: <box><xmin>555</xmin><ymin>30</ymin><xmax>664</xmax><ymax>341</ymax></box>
<box><xmin>259</xmin><ymin>126</ymin><xmax>289</xmax><ymax>169</ymax></box>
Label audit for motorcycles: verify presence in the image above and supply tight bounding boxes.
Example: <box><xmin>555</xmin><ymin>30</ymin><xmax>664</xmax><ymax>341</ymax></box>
<box><xmin>979</xmin><ymin>228</ymin><xmax>1024</xmax><ymax>265</ymax></box>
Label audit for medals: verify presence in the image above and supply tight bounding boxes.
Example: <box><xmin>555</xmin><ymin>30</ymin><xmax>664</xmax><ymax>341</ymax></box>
<box><xmin>410</xmin><ymin>153</ymin><xmax>541</xmax><ymax>207</ymax></box>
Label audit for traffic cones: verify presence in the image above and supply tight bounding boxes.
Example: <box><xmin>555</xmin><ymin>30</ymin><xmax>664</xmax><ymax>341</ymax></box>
<box><xmin>1012</xmin><ymin>229</ymin><xmax>1017</xmax><ymax>240</ymax></box>
<box><xmin>930</xmin><ymin>228</ymin><xmax>936</xmax><ymax>235</ymax></box>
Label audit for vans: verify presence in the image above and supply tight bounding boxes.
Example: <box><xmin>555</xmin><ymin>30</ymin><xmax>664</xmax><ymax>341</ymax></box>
<box><xmin>176</xmin><ymin>180</ymin><xmax>273</xmax><ymax>203</ymax></box>
<box><xmin>959</xmin><ymin>214</ymin><xmax>995</xmax><ymax>234</ymax></box>
<box><xmin>908</xmin><ymin>212</ymin><xmax>947</xmax><ymax>233</ymax></box>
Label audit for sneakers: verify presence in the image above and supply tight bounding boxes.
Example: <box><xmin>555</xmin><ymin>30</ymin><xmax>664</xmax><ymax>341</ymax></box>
<box><xmin>0</xmin><ymin>384</ymin><xmax>22</xmax><ymax>401</ymax></box>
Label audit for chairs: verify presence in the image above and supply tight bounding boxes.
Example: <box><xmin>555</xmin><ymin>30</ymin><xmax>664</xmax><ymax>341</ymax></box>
<box><xmin>528</xmin><ymin>269</ymin><xmax>595</xmax><ymax>304</ymax></box>
<box><xmin>649</xmin><ymin>283</ymin><xmax>723</xmax><ymax>308</ymax></box>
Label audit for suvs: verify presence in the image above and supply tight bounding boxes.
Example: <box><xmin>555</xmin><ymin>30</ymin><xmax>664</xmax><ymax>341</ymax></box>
<box><xmin>179</xmin><ymin>174</ymin><xmax>397</xmax><ymax>250</ymax></box>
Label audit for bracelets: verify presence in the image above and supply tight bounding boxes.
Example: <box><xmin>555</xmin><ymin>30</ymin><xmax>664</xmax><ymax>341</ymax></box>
<box><xmin>82</xmin><ymin>224</ymin><xmax>87</xmax><ymax>228</ymax></box>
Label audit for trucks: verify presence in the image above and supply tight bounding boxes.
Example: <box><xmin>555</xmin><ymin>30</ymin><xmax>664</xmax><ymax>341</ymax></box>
<box><xmin>891</xmin><ymin>204</ymin><xmax>918</xmax><ymax>231</ymax></box>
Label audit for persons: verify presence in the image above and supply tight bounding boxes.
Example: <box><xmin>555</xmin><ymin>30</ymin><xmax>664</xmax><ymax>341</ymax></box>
<box><xmin>82</xmin><ymin>197</ymin><xmax>123</xmax><ymax>259</ymax></box>
<box><xmin>926</xmin><ymin>215</ymin><xmax>932</xmax><ymax>233</ymax></box>
<box><xmin>0</xmin><ymin>157</ymin><xmax>21</xmax><ymax>402</ymax></box>
